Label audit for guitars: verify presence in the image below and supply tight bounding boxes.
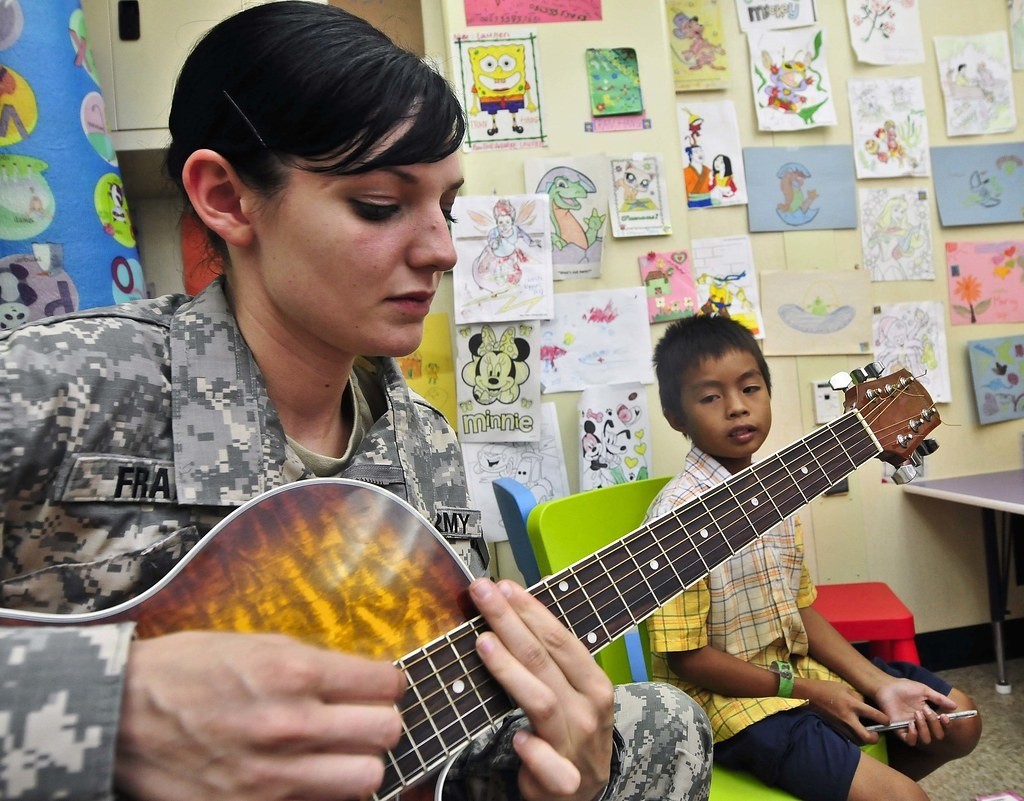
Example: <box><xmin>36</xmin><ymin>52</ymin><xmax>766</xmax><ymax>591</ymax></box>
<box><xmin>0</xmin><ymin>358</ymin><xmax>946</xmax><ymax>801</ymax></box>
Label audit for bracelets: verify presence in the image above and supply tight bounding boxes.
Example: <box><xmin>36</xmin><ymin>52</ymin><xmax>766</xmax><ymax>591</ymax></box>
<box><xmin>769</xmin><ymin>659</ymin><xmax>794</xmax><ymax>698</ymax></box>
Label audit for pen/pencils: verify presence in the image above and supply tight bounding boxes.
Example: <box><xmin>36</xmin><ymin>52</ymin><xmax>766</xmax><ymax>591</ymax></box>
<box><xmin>866</xmin><ymin>710</ymin><xmax>977</xmax><ymax>732</ymax></box>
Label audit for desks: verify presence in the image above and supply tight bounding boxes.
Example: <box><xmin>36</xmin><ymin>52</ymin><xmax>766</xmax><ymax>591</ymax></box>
<box><xmin>905</xmin><ymin>468</ymin><xmax>1024</xmax><ymax>694</ymax></box>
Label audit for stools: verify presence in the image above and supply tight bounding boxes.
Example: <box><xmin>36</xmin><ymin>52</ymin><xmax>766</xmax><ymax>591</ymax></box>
<box><xmin>808</xmin><ymin>581</ymin><xmax>921</xmax><ymax>667</ymax></box>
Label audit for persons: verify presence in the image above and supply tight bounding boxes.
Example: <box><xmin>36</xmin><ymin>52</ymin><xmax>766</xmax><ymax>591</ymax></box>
<box><xmin>0</xmin><ymin>0</ymin><xmax>715</xmax><ymax>801</ymax></box>
<box><xmin>640</xmin><ymin>311</ymin><xmax>985</xmax><ymax>801</ymax></box>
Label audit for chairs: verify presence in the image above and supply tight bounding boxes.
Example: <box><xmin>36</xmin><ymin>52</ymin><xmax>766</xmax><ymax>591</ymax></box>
<box><xmin>525</xmin><ymin>474</ymin><xmax>890</xmax><ymax>801</ymax></box>
<box><xmin>493</xmin><ymin>476</ymin><xmax>648</xmax><ymax>683</ymax></box>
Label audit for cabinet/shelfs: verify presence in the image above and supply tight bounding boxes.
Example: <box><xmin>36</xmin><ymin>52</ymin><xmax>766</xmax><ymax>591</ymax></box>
<box><xmin>80</xmin><ymin>0</ymin><xmax>328</xmax><ymax>199</ymax></box>
